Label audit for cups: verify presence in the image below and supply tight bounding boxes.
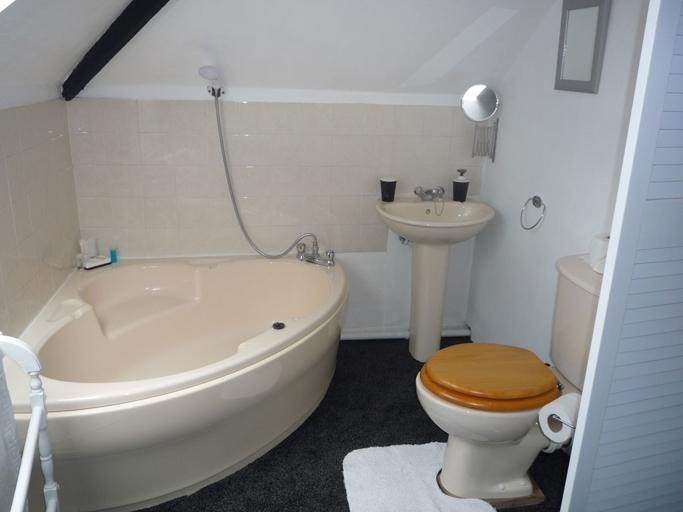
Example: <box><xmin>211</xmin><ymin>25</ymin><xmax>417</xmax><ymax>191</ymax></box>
<box><xmin>380</xmin><ymin>177</ymin><xmax>397</xmax><ymax>202</ymax></box>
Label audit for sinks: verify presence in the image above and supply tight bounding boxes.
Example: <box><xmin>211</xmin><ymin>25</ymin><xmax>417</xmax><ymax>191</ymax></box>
<box><xmin>377</xmin><ymin>195</ymin><xmax>494</xmax><ymax>245</ymax></box>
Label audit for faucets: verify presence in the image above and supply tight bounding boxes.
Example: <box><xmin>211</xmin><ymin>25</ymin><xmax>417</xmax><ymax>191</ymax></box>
<box><xmin>413</xmin><ymin>185</ymin><xmax>445</xmax><ymax>201</ymax></box>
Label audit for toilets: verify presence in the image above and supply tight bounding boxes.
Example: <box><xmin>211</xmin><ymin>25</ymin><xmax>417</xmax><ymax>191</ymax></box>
<box><xmin>416</xmin><ymin>256</ymin><xmax>604</xmax><ymax>498</ymax></box>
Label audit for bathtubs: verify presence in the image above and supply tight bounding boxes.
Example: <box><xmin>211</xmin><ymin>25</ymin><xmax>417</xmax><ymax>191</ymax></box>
<box><xmin>0</xmin><ymin>253</ymin><xmax>348</xmax><ymax>511</ymax></box>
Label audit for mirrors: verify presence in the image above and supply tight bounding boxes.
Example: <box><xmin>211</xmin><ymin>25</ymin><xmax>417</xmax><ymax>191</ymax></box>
<box><xmin>461</xmin><ymin>82</ymin><xmax>500</xmax><ymax>123</ymax></box>
<box><xmin>554</xmin><ymin>0</ymin><xmax>612</xmax><ymax>95</ymax></box>
